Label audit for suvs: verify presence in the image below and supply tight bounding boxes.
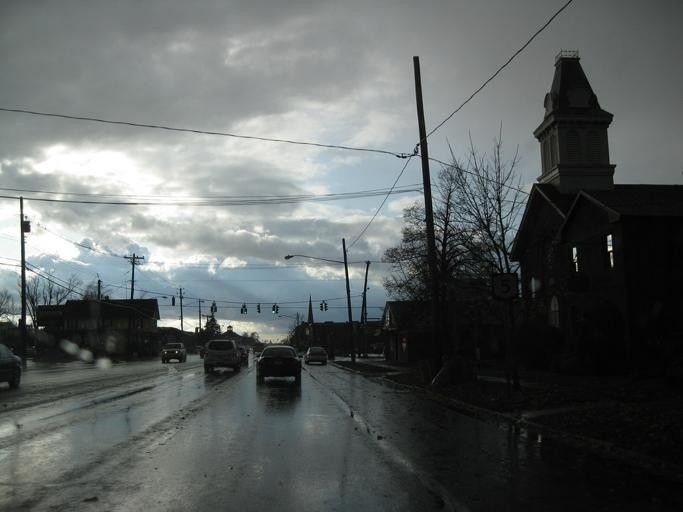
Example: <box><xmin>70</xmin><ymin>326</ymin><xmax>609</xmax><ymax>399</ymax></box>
<box><xmin>160</xmin><ymin>342</ymin><xmax>186</xmax><ymax>363</ymax></box>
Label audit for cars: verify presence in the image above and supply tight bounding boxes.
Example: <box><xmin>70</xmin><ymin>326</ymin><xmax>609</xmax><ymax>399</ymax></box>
<box><xmin>238</xmin><ymin>346</ymin><xmax>249</xmax><ymax>367</ymax></box>
<box><xmin>254</xmin><ymin>345</ymin><xmax>302</xmax><ymax>385</ymax></box>
<box><xmin>303</xmin><ymin>346</ymin><xmax>327</xmax><ymax>365</ymax></box>
<box><xmin>0</xmin><ymin>343</ymin><xmax>22</xmax><ymax>388</ymax></box>
<box><xmin>195</xmin><ymin>345</ymin><xmax>203</xmax><ymax>353</ymax></box>
<box><xmin>253</xmin><ymin>345</ymin><xmax>263</xmax><ymax>360</ymax></box>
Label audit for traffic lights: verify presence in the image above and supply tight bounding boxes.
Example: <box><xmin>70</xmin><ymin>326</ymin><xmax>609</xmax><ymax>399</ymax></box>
<box><xmin>210</xmin><ymin>306</ymin><xmax>213</xmax><ymax>312</ymax></box>
<box><xmin>271</xmin><ymin>305</ymin><xmax>275</xmax><ymax>314</ymax></box>
<box><xmin>319</xmin><ymin>302</ymin><xmax>323</xmax><ymax>311</ymax></box>
<box><xmin>256</xmin><ymin>304</ymin><xmax>260</xmax><ymax>313</ymax></box>
<box><xmin>240</xmin><ymin>308</ymin><xmax>243</xmax><ymax>314</ymax></box>
<box><xmin>213</xmin><ymin>306</ymin><xmax>217</xmax><ymax>312</ymax></box>
<box><xmin>324</xmin><ymin>302</ymin><xmax>328</xmax><ymax>312</ymax></box>
<box><xmin>275</xmin><ymin>305</ymin><xmax>279</xmax><ymax>313</ymax></box>
<box><xmin>244</xmin><ymin>308</ymin><xmax>247</xmax><ymax>314</ymax></box>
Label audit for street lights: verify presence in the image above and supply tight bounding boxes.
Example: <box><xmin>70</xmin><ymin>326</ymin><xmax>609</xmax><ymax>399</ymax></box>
<box><xmin>284</xmin><ymin>238</ymin><xmax>356</xmax><ymax>369</ymax></box>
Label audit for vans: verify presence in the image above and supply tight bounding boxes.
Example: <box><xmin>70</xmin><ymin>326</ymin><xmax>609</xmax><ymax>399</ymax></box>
<box><xmin>203</xmin><ymin>339</ymin><xmax>241</xmax><ymax>373</ymax></box>
